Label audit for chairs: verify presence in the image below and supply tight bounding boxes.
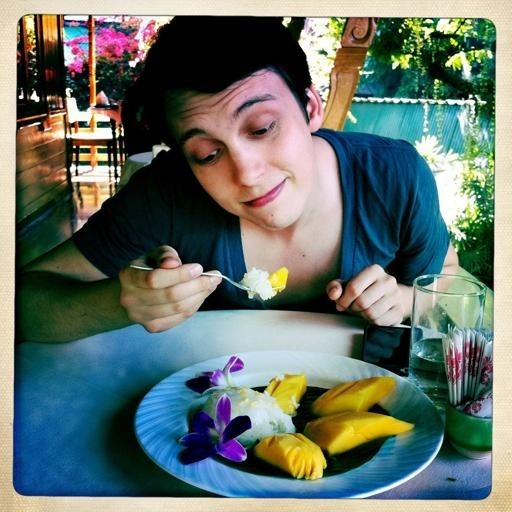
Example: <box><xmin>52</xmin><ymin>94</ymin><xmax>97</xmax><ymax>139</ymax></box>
<box><xmin>65</xmin><ymin>97</ymin><xmax>125</xmax><ymax>177</ymax></box>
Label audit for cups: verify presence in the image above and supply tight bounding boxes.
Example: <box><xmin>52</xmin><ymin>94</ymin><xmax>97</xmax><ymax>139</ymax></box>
<box><xmin>408</xmin><ymin>273</ymin><xmax>487</xmax><ymax>410</ymax></box>
<box><xmin>447</xmin><ymin>400</ymin><xmax>492</xmax><ymax>460</ymax></box>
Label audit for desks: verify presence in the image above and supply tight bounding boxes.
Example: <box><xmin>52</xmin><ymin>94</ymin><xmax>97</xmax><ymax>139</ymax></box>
<box><xmin>93</xmin><ymin>103</ymin><xmax>123</xmax><ymax>180</ymax></box>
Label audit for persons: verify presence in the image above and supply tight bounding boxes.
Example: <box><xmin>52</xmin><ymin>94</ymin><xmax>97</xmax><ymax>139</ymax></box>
<box><xmin>17</xmin><ymin>16</ymin><xmax>458</xmax><ymax>344</ymax></box>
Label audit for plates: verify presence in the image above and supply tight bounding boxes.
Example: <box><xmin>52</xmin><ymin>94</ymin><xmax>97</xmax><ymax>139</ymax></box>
<box><xmin>134</xmin><ymin>349</ymin><xmax>445</xmax><ymax>498</ymax></box>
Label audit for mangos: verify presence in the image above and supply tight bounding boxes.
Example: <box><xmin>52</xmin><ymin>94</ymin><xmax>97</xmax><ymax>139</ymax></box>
<box><xmin>255</xmin><ymin>374</ymin><xmax>416</xmax><ymax>482</ymax></box>
<box><xmin>270</xmin><ymin>268</ymin><xmax>289</xmax><ymax>292</ymax></box>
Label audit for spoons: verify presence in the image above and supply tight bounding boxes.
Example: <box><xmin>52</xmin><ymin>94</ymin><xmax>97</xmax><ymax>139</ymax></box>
<box><xmin>130</xmin><ymin>263</ymin><xmax>284</xmax><ymax>292</ymax></box>
